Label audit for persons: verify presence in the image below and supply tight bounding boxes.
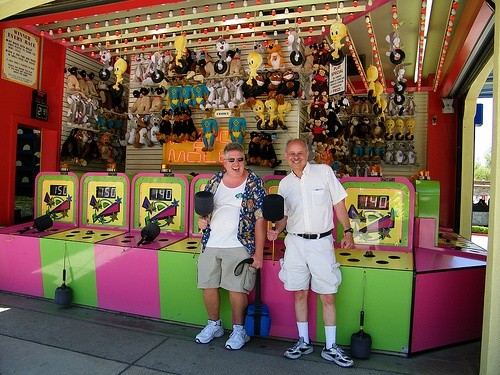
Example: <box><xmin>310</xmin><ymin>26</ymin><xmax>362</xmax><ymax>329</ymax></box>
<box><xmin>195</xmin><ymin>144</ymin><xmax>268</xmax><ymax>350</ymax></box>
<box><xmin>267</xmin><ymin>138</ymin><xmax>355</xmax><ymax>367</ymax></box>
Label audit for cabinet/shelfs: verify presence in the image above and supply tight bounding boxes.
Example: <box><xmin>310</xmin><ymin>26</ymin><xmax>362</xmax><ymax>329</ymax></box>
<box><xmin>38</xmin><ymin>0</ymin><xmax>459</xmax><ymax>180</ymax></box>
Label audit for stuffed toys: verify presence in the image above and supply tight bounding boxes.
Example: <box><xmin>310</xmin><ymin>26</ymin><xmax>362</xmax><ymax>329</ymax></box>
<box><xmin>59</xmin><ymin>21</ymin><xmax>418</xmax><ymax>177</ymax></box>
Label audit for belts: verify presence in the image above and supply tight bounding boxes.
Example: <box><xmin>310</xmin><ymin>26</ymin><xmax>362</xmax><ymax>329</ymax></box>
<box><xmin>296</xmin><ymin>229</ymin><xmax>332</xmax><ymax>239</ymax></box>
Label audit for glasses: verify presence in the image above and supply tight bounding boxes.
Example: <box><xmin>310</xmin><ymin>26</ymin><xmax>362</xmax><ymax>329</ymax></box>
<box><xmin>225</xmin><ymin>157</ymin><xmax>244</xmax><ymax>162</ymax></box>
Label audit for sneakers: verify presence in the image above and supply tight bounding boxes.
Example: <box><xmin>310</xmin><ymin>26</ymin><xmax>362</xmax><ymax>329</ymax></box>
<box><xmin>321</xmin><ymin>343</ymin><xmax>354</xmax><ymax>367</ymax></box>
<box><xmin>224</xmin><ymin>324</ymin><xmax>250</xmax><ymax>350</ymax></box>
<box><xmin>283</xmin><ymin>336</ymin><xmax>314</xmax><ymax>360</ymax></box>
<box><xmin>194</xmin><ymin>318</ymin><xmax>224</xmax><ymax>343</ymax></box>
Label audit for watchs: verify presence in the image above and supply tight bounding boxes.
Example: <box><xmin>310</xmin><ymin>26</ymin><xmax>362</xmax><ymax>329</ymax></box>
<box><xmin>344</xmin><ymin>228</ymin><xmax>354</xmax><ymax>233</ymax></box>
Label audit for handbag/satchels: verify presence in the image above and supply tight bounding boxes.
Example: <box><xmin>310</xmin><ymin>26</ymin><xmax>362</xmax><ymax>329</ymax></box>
<box><xmin>245</xmin><ymin>304</ymin><xmax>272</xmax><ymax>337</ymax></box>
